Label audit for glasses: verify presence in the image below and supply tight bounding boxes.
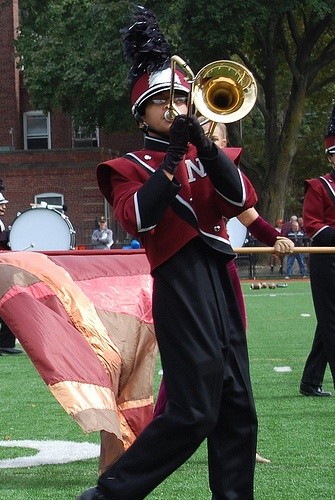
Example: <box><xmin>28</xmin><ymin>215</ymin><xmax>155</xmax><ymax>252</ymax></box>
<box><xmin>150</xmin><ymin>94</ymin><xmax>189</xmax><ymax>105</ymax></box>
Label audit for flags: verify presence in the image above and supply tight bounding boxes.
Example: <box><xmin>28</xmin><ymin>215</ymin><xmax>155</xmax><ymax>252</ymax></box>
<box><xmin>0</xmin><ymin>249</ymin><xmax>158</xmax><ymax>477</ymax></box>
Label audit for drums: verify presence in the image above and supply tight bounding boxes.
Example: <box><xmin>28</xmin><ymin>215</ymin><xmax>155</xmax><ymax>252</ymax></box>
<box><xmin>8</xmin><ymin>206</ymin><xmax>77</xmax><ymax>251</ymax></box>
<box><xmin>226</xmin><ymin>217</ymin><xmax>251</xmax><ymax>247</ymax></box>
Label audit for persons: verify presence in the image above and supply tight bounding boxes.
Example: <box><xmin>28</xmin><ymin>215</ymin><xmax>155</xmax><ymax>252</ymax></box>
<box><xmin>75</xmin><ymin>68</ymin><xmax>258</xmax><ymax>500</ymax></box>
<box><xmin>285</xmin><ymin>221</ymin><xmax>308</xmax><ymax>278</ymax></box>
<box><xmin>92</xmin><ymin>216</ymin><xmax>114</xmax><ymax>250</ymax></box>
<box><xmin>268</xmin><ymin>215</ymin><xmax>305</xmax><ymax>279</ymax></box>
<box><xmin>153</xmin><ymin>116</ymin><xmax>294</xmax><ymax>463</ymax></box>
<box><xmin>299</xmin><ymin>135</ymin><xmax>335</xmax><ymax>397</ymax></box>
<box><xmin>0</xmin><ymin>192</ymin><xmax>24</xmax><ymax>356</ymax></box>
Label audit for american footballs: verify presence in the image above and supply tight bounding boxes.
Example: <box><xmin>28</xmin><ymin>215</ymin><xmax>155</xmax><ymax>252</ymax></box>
<box><xmin>251</xmin><ymin>282</ymin><xmax>275</xmax><ymax>289</ymax></box>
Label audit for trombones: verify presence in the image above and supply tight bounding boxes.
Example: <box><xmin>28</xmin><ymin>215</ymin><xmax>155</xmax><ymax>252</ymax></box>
<box><xmin>164</xmin><ymin>55</ymin><xmax>258</xmax><ymax>140</ymax></box>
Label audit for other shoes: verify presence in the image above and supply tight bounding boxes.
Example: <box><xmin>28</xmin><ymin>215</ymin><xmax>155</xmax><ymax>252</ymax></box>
<box><xmin>77</xmin><ymin>487</ymin><xmax>105</xmax><ymax>500</ymax></box>
<box><xmin>256</xmin><ymin>452</ymin><xmax>271</xmax><ymax>463</ymax></box>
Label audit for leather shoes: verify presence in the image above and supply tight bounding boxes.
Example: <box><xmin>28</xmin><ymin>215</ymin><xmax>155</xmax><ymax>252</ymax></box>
<box><xmin>0</xmin><ymin>348</ymin><xmax>23</xmax><ymax>356</ymax></box>
<box><xmin>299</xmin><ymin>383</ymin><xmax>331</xmax><ymax>397</ymax></box>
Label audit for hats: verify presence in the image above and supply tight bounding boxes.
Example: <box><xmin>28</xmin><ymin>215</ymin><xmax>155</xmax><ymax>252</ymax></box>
<box><xmin>324</xmin><ymin>103</ymin><xmax>335</xmax><ymax>155</ymax></box>
<box><xmin>98</xmin><ymin>216</ymin><xmax>106</xmax><ymax>222</ymax></box>
<box><xmin>119</xmin><ymin>3</ymin><xmax>192</xmax><ymax>116</ymax></box>
<box><xmin>0</xmin><ymin>179</ymin><xmax>9</xmax><ymax>204</ymax></box>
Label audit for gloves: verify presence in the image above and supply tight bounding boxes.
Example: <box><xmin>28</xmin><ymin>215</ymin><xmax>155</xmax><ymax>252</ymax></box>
<box><xmin>187</xmin><ymin>117</ymin><xmax>210</xmax><ymax>153</ymax></box>
<box><xmin>160</xmin><ymin>115</ymin><xmax>189</xmax><ymax>175</ymax></box>
